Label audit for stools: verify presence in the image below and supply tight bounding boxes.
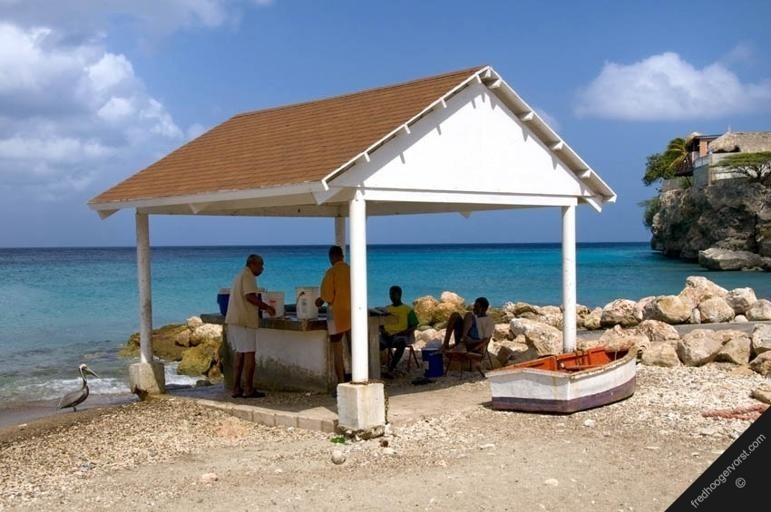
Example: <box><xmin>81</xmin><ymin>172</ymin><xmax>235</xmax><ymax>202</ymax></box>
<box><xmin>385</xmin><ymin>344</ymin><xmax>420</xmax><ymax>372</ymax></box>
<box><xmin>442</xmin><ymin>352</ymin><xmax>486</xmax><ymax>381</ymax></box>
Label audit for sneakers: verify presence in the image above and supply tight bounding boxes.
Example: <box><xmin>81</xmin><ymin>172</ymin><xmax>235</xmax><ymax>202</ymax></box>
<box><xmin>384</xmin><ymin>371</ymin><xmax>393</xmax><ymax>380</ymax></box>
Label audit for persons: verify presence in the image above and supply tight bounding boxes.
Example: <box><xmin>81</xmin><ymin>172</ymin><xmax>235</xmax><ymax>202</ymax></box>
<box><xmin>435</xmin><ymin>295</ymin><xmax>498</xmax><ymax>355</ymax></box>
<box><xmin>378</xmin><ymin>284</ymin><xmax>420</xmax><ymax>378</ymax></box>
<box><xmin>314</xmin><ymin>245</ymin><xmax>351</xmax><ymax>397</ymax></box>
<box><xmin>224</xmin><ymin>253</ymin><xmax>276</xmax><ymax>399</ymax></box>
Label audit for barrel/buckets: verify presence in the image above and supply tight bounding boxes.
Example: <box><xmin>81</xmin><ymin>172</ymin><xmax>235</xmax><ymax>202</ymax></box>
<box><xmin>295</xmin><ymin>286</ymin><xmax>319</xmax><ymax>320</ymax></box>
<box><xmin>215</xmin><ymin>287</ymin><xmax>230</xmax><ymax>316</ymax></box>
<box><xmin>422</xmin><ymin>346</ymin><xmax>445</xmax><ymax>378</ymax></box>
<box><xmin>261</xmin><ymin>291</ymin><xmax>286</xmax><ymax>318</ymax></box>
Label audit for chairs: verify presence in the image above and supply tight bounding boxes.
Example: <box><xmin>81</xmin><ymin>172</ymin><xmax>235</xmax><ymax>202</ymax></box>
<box><xmin>469</xmin><ymin>335</ymin><xmax>494</xmax><ymax>372</ymax></box>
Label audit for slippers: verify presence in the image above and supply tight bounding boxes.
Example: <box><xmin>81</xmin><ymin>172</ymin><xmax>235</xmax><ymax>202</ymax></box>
<box><xmin>411</xmin><ymin>376</ymin><xmax>433</xmax><ymax>385</ymax></box>
<box><xmin>232</xmin><ymin>388</ymin><xmax>265</xmax><ymax>399</ymax></box>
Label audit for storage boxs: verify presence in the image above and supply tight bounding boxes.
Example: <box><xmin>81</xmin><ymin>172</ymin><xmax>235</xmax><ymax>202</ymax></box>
<box><xmin>217</xmin><ymin>287</ymin><xmax>265</xmax><ymax>316</ymax></box>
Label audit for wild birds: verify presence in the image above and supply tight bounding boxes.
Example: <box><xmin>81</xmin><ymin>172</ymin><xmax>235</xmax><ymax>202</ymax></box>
<box><xmin>57</xmin><ymin>363</ymin><xmax>101</xmax><ymax>413</ymax></box>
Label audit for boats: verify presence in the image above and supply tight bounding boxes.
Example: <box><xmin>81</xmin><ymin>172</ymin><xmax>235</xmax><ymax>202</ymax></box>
<box><xmin>485</xmin><ymin>344</ymin><xmax>639</xmax><ymax>415</ymax></box>
